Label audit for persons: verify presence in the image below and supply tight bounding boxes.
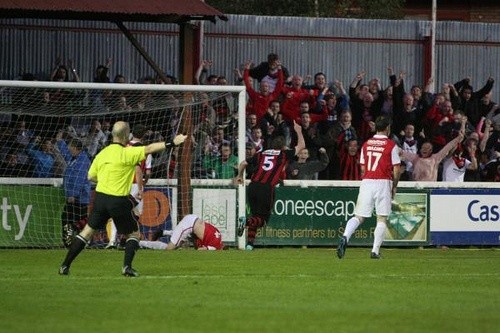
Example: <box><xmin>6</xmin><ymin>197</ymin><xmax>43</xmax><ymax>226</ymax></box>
<box><xmin>60</xmin><ymin>121</ymin><xmax>187</xmax><ymax>277</ymax></box>
<box><xmin>191</xmin><ymin>53</ymin><xmax>500</xmax><ymax>182</ymax></box>
<box><xmin>337</xmin><ymin>118</ymin><xmax>401</xmax><ymax>258</ymax></box>
<box><xmin>0</xmin><ymin>58</ymin><xmax>181</xmax><ymax>179</ymax></box>
<box><xmin>106</xmin><ymin>128</ymin><xmax>151</xmax><ymax>250</ymax></box>
<box><xmin>138</xmin><ymin>215</ymin><xmax>224</xmax><ymax>251</ymax></box>
<box><xmin>57</xmin><ymin>133</ymin><xmax>93</xmax><ymax>247</ymax></box>
<box><xmin>237</xmin><ymin>119</ymin><xmax>305</xmax><ymax>250</ymax></box>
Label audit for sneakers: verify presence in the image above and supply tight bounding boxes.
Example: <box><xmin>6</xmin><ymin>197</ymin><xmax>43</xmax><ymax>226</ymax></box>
<box><xmin>370</xmin><ymin>252</ymin><xmax>381</xmax><ymax>260</ymax></box>
<box><xmin>237</xmin><ymin>216</ymin><xmax>246</xmax><ymax>236</ymax></box>
<box><xmin>337</xmin><ymin>236</ymin><xmax>347</xmax><ymax>259</ymax></box>
<box><xmin>245</xmin><ymin>245</ymin><xmax>253</xmax><ymax>251</ymax></box>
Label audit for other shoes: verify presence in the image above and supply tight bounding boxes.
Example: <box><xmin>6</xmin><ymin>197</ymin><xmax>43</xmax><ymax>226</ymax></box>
<box><xmin>152</xmin><ymin>229</ymin><xmax>163</xmax><ymax>242</ymax></box>
<box><xmin>105</xmin><ymin>244</ymin><xmax>117</xmax><ymax>249</ymax></box>
<box><xmin>59</xmin><ymin>266</ymin><xmax>68</xmax><ymax>275</ymax></box>
<box><xmin>122</xmin><ymin>266</ymin><xmax>137</xmax><ymax>277</ymax></box>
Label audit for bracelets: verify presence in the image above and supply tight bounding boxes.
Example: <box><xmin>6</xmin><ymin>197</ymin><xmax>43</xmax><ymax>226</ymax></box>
<box><xmin>165</xmin><ymin>140</ymin><xmax>175</xmax><ymax>148</ymax></box>
